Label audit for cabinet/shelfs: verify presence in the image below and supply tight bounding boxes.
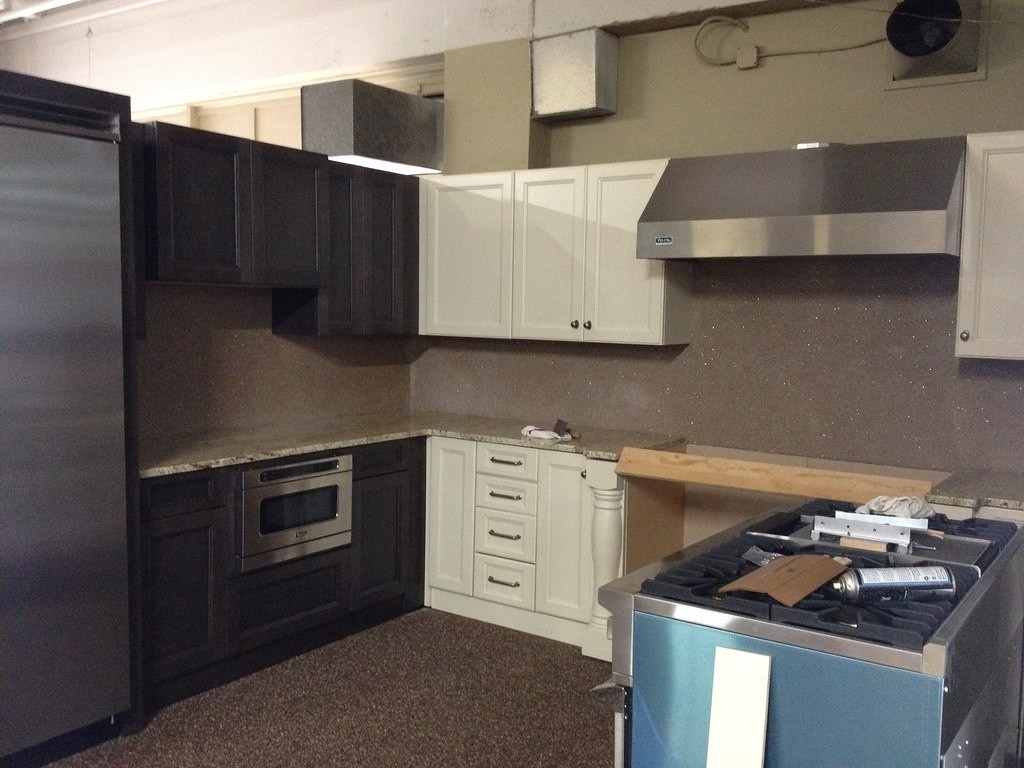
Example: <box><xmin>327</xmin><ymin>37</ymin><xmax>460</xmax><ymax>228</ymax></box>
<box><xmin>122</xmin><ymin>410</ymin><xmax>625</xmax><ymax>707</ymax></box>
<box><xmin>419</xmin><ymin>171</ymin><xmax>514</xmax><ymax>340</ymax></box>
<box><xmin>513</xmin><ymin>157</ymin><xmax>691</xmax><ymax>346</ymax></box>
<box><xmin>139</xmin><ymin>121</ymin><xmax>329</xmax><ymax>286</ymax></box>
<box><xmin>271</xmin><ymin>156</ymin><xmax>419</xmax><ymax>336</ymax></box>
<box><xmin>955</xmin><ymin>129</ymin><xmax>1024</xmax><ymax>360</ymax></box>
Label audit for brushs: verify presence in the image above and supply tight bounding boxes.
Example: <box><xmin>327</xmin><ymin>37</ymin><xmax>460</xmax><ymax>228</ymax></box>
<box><xmin>553</xmin><ymin>418</ymin><xmax>581</xmax><ymax>439</ymax></box>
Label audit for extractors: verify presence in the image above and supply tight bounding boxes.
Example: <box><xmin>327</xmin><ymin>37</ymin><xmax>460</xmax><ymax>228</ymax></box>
<box><xmin>637</xmin><ymin>137</ymin><xmax>967</xmax><ymax>264</ymax></box>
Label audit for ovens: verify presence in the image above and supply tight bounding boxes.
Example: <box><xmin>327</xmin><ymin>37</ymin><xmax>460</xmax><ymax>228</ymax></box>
<box><xmin>230</xmin><ymin>454</ymin><xmax>352</xmax><ymax>572</ymax></box>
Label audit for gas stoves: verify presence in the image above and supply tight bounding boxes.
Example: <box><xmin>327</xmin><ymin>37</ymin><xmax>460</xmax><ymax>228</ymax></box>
<box><xmin>597</xmin><ymin>499</ymin><xmax>1024</xmax><ymax>767</ymax></box>
